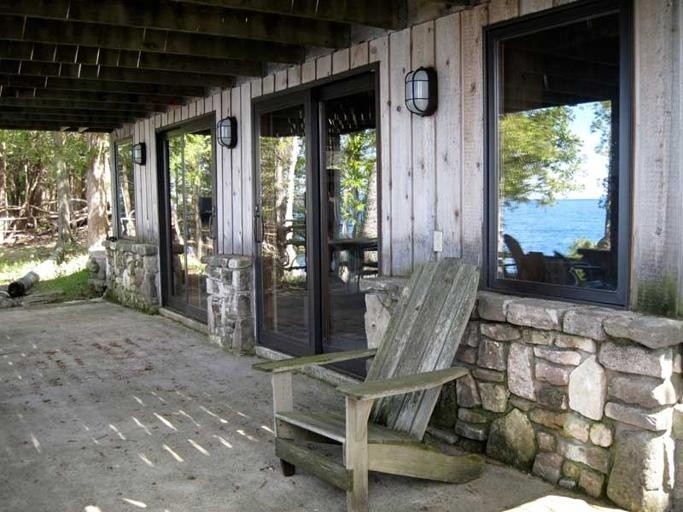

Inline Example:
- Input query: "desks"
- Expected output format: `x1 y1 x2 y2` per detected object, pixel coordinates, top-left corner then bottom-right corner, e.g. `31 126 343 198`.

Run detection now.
329 238 378 295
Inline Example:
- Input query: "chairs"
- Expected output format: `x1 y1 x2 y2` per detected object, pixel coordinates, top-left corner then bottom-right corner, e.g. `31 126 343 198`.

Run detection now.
503 233 577 287
273 217 307 290
250 255 487 512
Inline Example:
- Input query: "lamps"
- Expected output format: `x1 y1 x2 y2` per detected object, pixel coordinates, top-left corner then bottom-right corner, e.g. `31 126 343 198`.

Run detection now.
404 63 438 117
132 141 146 165
217 115 238 149
